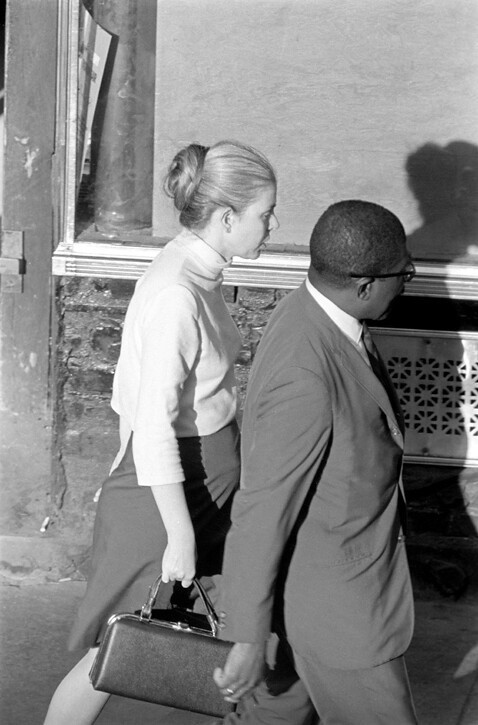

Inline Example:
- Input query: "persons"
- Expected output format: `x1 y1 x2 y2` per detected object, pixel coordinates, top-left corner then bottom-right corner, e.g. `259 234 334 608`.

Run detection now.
40 137 278 725
213 200 420 725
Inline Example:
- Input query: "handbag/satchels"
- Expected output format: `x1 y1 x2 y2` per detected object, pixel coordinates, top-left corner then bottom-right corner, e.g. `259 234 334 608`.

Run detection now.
87 573 237 719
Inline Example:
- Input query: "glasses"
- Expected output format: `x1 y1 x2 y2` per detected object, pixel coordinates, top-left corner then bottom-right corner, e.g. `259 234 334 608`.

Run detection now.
349 260 416 281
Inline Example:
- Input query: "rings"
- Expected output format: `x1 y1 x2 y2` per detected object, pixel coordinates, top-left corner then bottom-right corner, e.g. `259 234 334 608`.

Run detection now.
226 688 234 695
